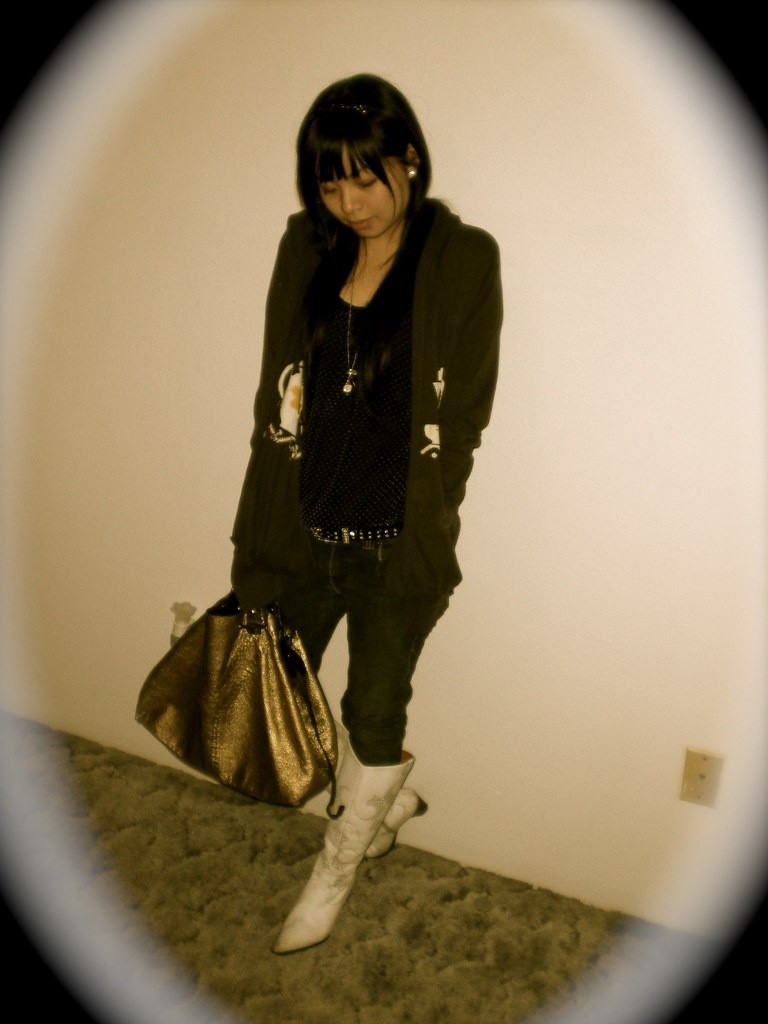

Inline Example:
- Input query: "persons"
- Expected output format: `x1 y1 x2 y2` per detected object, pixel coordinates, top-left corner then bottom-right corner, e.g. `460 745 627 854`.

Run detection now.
230 73 503 954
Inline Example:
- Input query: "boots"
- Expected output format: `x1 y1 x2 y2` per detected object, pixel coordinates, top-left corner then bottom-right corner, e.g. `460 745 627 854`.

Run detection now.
333 751 429 857
273 734 415 954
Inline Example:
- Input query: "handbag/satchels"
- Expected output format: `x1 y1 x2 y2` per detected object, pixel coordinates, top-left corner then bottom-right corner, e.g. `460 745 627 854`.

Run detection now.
135 591 339 808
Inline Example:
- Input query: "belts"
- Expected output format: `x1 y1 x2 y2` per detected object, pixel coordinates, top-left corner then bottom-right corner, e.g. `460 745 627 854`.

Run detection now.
306 526 401 544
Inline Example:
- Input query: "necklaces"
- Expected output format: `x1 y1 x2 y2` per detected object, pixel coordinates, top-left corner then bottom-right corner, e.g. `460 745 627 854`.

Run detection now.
343 270 358 394
